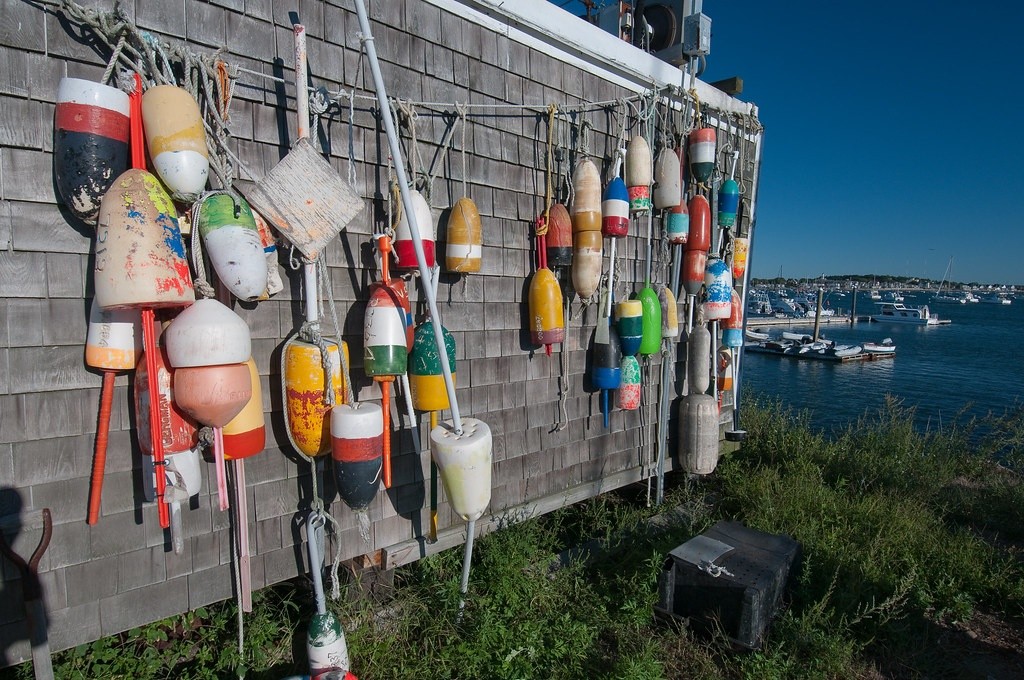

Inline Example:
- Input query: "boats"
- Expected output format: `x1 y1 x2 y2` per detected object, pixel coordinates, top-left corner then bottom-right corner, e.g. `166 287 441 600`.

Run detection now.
872 302 938 326
745 329 896 357
983 291 1012 305
748 282 982 318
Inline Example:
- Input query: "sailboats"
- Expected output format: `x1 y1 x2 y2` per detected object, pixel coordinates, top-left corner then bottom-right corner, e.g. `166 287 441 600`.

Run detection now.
929 256 965 304
